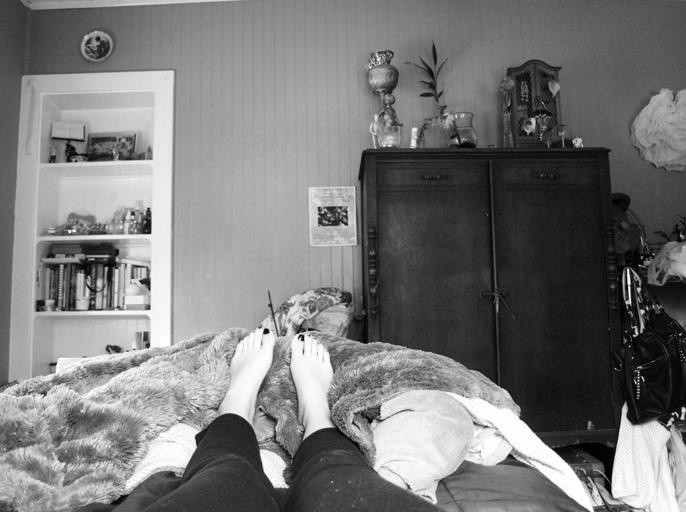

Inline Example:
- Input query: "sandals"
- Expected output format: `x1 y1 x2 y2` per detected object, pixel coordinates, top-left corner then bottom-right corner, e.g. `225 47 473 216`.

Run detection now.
574 468 607 512
589 469 629 512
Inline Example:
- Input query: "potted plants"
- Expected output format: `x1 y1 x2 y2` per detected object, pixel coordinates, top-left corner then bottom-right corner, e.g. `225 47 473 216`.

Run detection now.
416 111 452 148
401 40 477 149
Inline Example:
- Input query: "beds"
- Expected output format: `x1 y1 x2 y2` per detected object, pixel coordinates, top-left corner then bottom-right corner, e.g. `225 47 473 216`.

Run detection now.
2 326 582 511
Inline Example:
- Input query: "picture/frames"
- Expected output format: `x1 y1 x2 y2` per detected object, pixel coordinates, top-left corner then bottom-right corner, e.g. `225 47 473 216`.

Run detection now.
87 132 139 161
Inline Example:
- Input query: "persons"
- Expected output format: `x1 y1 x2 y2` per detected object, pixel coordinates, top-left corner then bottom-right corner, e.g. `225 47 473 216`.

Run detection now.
369 113 382 149
140 324 446 512
519 121 534 136
377 93 404 132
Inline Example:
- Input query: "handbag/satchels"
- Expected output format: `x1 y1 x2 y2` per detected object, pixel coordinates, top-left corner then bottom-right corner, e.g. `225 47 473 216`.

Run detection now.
614 263 686 425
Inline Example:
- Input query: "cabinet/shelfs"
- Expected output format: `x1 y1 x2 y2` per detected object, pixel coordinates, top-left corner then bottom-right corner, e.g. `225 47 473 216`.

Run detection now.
8 70 174 384
356 148 625 448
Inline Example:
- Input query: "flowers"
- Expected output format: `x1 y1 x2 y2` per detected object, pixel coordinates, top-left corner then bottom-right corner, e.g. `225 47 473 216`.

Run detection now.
628 87 686 173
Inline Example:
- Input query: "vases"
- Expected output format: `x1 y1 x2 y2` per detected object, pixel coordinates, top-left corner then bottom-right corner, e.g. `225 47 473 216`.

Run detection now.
409 127 427 149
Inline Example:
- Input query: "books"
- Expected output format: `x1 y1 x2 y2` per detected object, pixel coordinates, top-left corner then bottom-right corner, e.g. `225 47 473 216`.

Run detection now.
45 262 151 311
